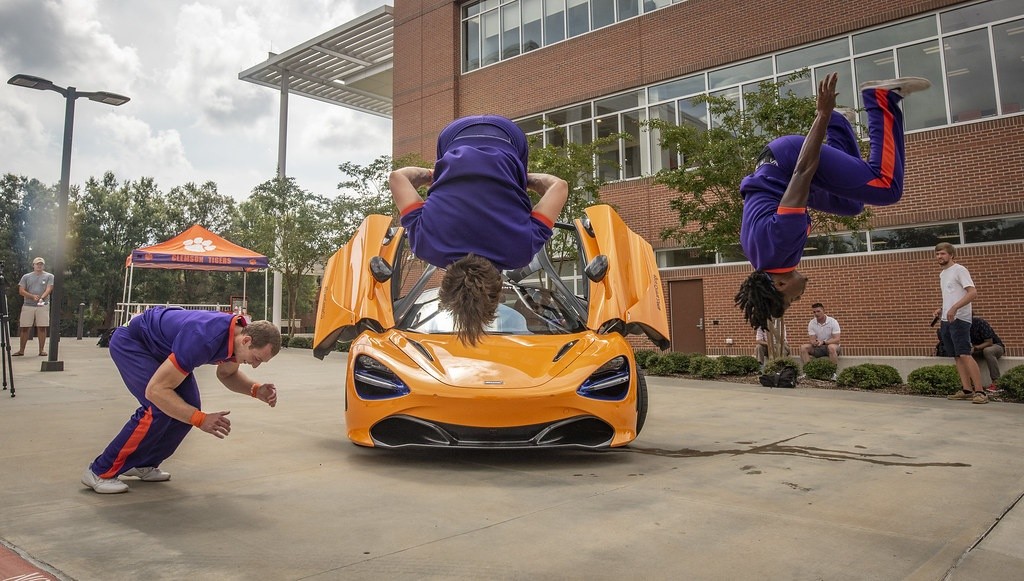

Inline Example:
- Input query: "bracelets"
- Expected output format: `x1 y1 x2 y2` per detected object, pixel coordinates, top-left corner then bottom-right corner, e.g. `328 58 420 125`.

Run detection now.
41 297 45 300
250 383 260 399
189 409 207 428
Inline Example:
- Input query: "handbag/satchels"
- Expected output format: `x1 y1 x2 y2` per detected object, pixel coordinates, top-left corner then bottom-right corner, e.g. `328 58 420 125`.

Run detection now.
759 365 798 387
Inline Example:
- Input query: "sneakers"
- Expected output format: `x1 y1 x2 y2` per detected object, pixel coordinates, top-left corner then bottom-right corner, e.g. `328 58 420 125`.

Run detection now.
814 107 857 122
121 466 171 481
79 462 129 493
947 389 975 399
859 77 931 98
972 391 989 404
986 383 1004 392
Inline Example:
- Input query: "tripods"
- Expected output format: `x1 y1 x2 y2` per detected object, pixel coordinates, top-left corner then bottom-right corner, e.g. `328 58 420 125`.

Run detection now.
0 260 15 397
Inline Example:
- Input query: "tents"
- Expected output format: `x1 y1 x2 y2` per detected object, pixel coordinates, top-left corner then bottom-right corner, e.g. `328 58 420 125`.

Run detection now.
120 226 270 326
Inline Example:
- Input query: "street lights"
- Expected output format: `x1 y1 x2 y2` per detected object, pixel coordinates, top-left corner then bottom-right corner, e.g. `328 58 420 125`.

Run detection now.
7 75 131 375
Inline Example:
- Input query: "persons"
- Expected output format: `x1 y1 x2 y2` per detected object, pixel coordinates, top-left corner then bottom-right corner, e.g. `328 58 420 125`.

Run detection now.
80 303 283 495
12 257 54 356
798 304 842 380
734 71 932 331
932 242 989 404
970 317 1006 392
388 113 569 349
756 319 792 365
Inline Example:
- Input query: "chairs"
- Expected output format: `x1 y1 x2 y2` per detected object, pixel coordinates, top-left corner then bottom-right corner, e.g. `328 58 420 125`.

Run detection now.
513 292 558 333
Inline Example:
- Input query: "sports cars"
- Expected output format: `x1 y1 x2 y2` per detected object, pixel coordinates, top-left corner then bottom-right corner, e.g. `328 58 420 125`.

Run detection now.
314 205 676 452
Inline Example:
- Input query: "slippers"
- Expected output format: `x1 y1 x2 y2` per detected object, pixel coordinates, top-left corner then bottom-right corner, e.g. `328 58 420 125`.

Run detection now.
38 351 47 356
11 351 24 356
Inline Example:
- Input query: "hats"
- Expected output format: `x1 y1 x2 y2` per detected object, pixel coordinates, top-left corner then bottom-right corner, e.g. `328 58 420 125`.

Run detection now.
32 257 45 264
